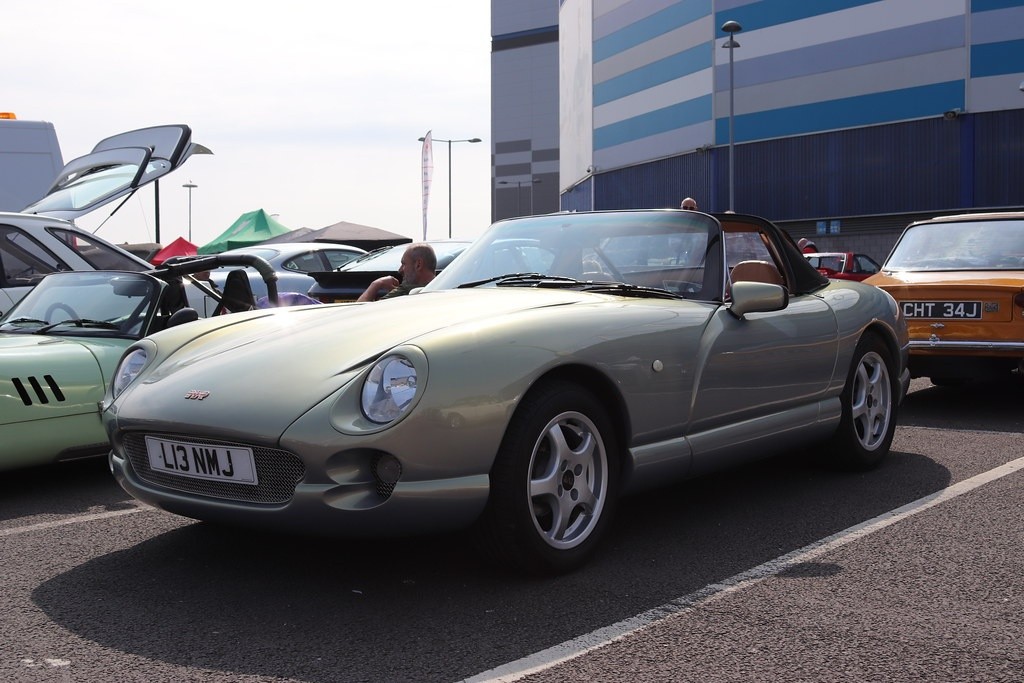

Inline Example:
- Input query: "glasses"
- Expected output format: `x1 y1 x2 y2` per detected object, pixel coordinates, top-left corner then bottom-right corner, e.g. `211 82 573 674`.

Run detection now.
683 206 694 210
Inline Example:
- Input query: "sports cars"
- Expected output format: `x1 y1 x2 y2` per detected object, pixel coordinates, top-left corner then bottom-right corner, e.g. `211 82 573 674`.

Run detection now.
0 110 779 486
96 207 912 584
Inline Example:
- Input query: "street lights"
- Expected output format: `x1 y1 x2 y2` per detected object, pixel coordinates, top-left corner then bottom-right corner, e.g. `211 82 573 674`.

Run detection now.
182 184 198 242
418 137 483 238
586 165 597 211
499 179 542 217
721 20 745 209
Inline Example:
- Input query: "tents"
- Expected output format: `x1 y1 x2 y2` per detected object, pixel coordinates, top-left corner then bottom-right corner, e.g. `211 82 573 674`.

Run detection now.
289 220 413 252
253 227 315 245
197 208 292 255
150 236 198 266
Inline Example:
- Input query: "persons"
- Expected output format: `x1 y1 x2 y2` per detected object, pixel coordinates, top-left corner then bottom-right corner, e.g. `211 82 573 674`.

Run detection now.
680 197 697 211
192 270 219 295
356 242 437 302
797 238 818 253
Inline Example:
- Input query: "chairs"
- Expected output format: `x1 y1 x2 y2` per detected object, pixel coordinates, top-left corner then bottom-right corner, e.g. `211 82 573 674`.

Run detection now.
731 260 783 295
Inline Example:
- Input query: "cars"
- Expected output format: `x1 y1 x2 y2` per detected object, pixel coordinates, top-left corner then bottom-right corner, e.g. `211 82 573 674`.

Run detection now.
860 211 1024 388
802 251 881 283
